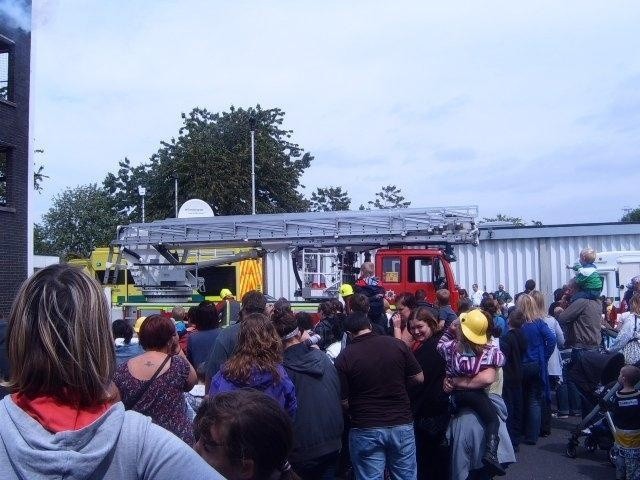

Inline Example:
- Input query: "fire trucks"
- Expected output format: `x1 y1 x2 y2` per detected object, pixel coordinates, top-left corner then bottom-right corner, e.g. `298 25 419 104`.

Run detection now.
100 202 480 337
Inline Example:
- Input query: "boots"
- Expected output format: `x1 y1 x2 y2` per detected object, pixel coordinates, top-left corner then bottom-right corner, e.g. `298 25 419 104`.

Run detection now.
481 433 506 476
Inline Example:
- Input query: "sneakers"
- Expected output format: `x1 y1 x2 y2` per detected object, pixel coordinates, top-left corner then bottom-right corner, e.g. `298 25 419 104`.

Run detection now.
551 413 569 419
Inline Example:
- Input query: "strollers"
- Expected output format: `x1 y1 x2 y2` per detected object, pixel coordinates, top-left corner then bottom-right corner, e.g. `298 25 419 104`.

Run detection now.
562 350 640 465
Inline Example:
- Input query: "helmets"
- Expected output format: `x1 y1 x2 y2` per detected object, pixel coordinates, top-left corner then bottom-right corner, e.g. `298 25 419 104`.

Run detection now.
220 289 232 299
339 284 354 297
134 316 147 333
460 309 488 345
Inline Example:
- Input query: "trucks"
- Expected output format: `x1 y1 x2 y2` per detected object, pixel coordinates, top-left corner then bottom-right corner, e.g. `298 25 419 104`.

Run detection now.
574 249 640 307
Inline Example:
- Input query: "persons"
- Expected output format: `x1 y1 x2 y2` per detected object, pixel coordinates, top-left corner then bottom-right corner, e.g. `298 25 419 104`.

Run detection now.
1 265 226 480
111 245 640 479
